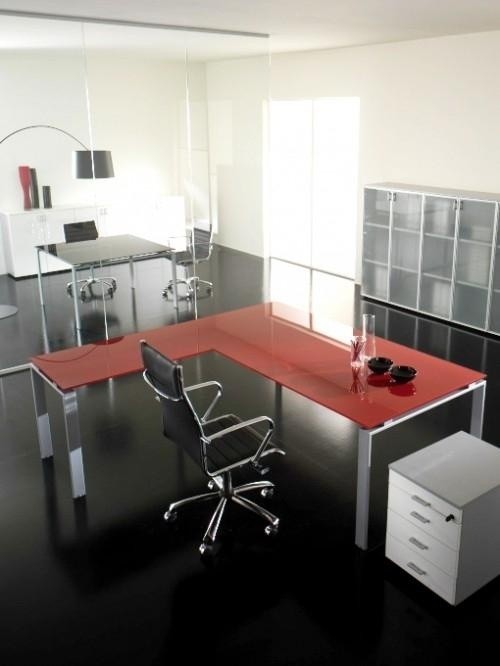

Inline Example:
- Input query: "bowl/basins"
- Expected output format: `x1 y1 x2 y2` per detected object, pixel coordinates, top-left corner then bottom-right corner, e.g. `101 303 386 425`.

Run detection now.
368 356 417 384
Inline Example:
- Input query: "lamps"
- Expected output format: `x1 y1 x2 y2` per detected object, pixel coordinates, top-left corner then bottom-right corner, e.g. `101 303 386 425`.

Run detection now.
0 125 113 179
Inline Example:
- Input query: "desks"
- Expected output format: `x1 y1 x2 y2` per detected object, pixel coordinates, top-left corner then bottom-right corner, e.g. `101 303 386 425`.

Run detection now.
29 302 487 549
35 234 179 354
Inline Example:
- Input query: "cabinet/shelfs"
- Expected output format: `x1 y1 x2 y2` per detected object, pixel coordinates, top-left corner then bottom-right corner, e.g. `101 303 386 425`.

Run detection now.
0 204 108 278
360 181 500 338
385 430 500 606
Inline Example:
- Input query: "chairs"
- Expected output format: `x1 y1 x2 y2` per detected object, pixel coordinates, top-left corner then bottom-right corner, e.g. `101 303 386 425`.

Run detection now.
63 221 116 302
140 340 286 555
161 224 214 302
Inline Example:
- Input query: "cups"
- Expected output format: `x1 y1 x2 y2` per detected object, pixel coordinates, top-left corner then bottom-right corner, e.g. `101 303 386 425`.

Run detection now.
350 314 375 367
